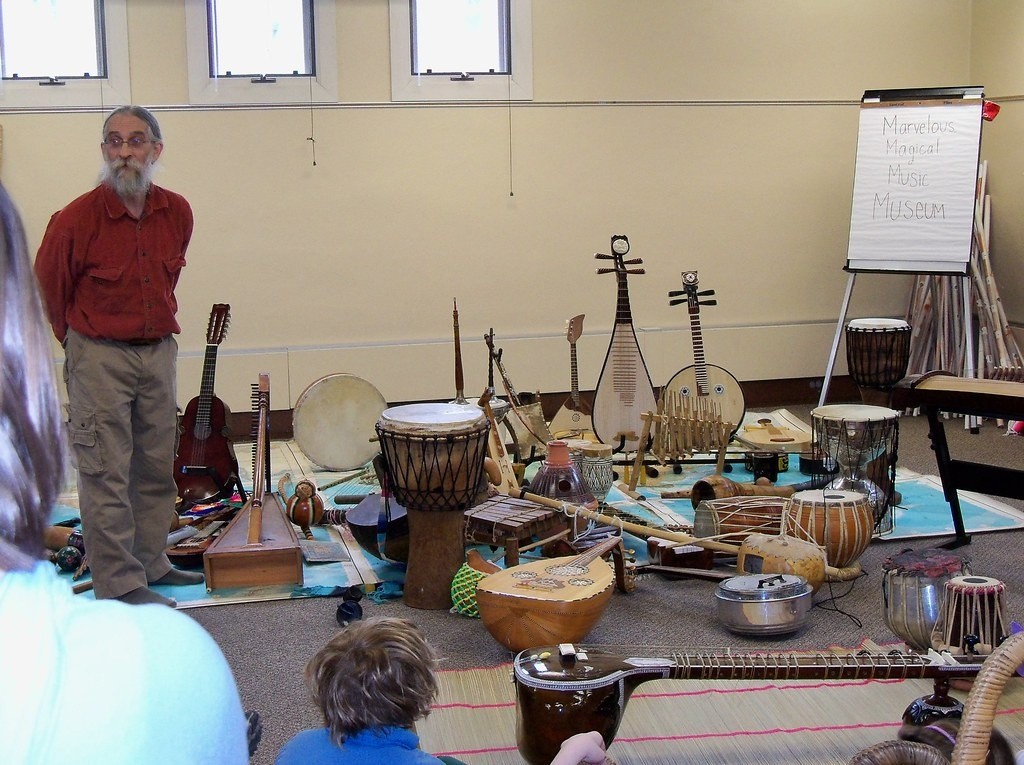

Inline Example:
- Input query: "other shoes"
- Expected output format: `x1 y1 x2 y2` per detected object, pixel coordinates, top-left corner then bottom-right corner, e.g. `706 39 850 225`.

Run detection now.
245 709 263 757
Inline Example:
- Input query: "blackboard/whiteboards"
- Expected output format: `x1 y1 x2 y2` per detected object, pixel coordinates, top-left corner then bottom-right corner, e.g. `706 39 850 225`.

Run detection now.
841 85 986 276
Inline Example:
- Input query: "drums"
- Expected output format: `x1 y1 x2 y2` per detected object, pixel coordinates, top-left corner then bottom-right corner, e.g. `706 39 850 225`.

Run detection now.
289 370 389 473
374 401 492 612
811 403 901 539
844 317 913 409
694 494 793 554
880 547 1012 695
787 487 876 568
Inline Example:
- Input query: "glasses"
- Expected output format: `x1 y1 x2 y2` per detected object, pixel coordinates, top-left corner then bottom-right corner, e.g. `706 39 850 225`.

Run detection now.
102 135 155 149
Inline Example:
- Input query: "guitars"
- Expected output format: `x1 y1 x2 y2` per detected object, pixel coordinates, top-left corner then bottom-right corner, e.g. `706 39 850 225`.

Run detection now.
173 299 240 504
547 312 594 436
474 535 640 657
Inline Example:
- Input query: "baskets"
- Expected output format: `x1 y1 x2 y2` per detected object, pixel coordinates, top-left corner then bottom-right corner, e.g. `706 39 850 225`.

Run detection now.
450 562 491 619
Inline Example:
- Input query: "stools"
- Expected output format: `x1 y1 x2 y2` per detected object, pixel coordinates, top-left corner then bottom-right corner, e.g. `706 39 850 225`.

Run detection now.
464 496 567 571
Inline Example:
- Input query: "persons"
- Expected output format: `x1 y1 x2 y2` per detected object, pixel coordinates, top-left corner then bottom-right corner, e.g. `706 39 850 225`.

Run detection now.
35 105 204 607
0 186 251 765
272 618 445 765
